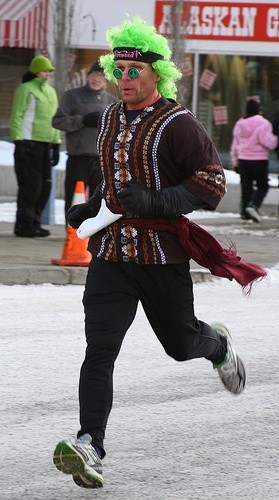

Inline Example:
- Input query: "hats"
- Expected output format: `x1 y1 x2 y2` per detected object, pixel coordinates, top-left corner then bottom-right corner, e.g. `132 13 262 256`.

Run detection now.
87 61 104 75
29 56 55 74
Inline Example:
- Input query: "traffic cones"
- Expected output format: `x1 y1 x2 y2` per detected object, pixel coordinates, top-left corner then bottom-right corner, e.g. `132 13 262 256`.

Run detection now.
52 180 93 267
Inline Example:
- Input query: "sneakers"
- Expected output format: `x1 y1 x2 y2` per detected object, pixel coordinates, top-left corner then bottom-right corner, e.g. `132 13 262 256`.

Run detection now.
53 433 104 489
212 322 246 395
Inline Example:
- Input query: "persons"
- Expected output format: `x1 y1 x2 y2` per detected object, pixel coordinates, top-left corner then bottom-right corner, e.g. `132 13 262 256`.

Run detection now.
51 19 247 490
230 93 278 224
8 55 120 239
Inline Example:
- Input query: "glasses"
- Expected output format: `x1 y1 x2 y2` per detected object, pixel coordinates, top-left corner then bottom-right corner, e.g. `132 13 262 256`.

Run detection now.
112 66 152 80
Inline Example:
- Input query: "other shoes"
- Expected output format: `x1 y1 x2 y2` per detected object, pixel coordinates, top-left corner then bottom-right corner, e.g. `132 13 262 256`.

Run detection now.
242 217 254 224
246 206 261 223
15 226 51 238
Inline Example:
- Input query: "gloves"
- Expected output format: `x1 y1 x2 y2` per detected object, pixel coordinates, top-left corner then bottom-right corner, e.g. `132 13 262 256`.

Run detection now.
235 167 241 173
65 196 100 229
117 181 162 217
50 144 59 166
14 141 26 162
83 111 101 125
